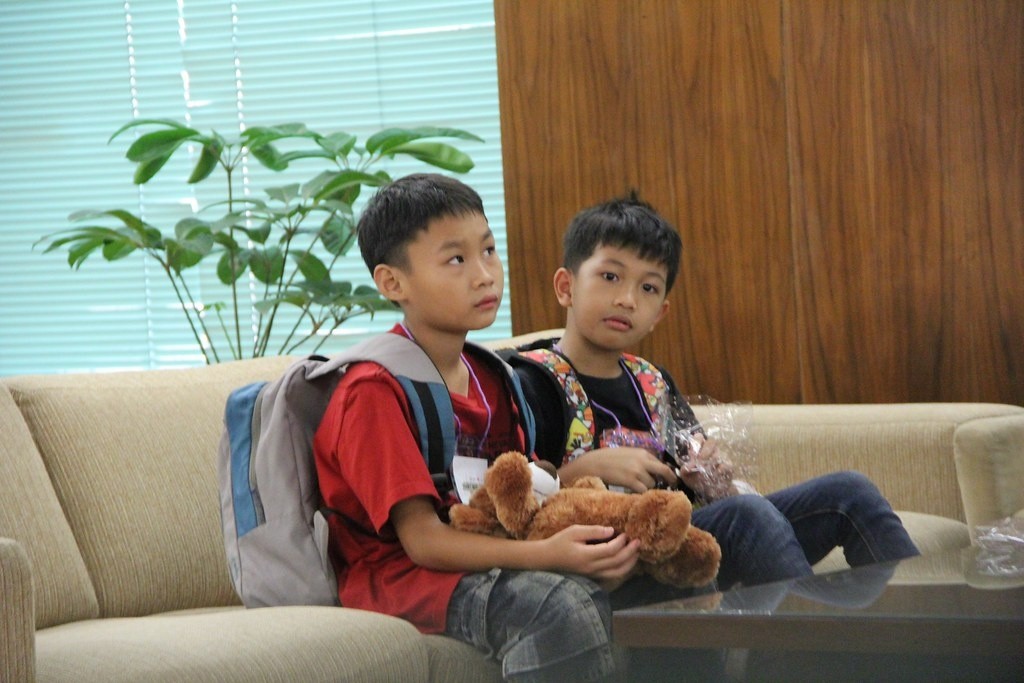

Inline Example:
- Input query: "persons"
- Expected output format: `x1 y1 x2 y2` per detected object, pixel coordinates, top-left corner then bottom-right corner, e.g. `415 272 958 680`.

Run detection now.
314 173 750 683
512 186 923 585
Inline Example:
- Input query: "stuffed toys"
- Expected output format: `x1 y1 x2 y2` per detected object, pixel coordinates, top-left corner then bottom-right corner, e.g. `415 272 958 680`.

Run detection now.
448 452 722 586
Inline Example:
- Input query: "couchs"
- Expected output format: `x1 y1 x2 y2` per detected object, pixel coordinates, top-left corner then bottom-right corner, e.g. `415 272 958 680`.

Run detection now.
1 328 1024 683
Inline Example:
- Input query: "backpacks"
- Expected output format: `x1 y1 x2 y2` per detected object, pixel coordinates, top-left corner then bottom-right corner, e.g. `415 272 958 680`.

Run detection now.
218 333 538 609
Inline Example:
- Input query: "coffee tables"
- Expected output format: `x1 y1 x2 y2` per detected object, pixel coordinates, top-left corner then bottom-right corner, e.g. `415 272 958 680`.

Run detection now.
613 555 1024 683
